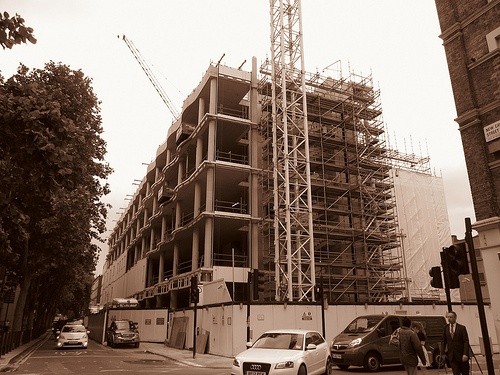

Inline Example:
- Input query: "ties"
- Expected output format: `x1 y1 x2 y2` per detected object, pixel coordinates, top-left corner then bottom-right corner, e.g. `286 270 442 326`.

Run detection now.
451 324 454 332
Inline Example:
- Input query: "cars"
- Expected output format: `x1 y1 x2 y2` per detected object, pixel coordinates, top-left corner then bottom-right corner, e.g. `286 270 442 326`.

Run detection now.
56 325 91 349
231 329 334 375
106 320 141 348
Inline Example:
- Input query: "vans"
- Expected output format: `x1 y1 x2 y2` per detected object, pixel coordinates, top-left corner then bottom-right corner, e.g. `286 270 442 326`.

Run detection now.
330 314 448 373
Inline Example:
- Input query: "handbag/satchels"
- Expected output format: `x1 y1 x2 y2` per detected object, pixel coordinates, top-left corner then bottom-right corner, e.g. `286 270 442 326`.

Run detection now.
388 328 400 346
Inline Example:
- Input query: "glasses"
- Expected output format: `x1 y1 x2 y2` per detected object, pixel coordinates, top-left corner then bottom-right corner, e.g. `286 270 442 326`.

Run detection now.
446 315 453 318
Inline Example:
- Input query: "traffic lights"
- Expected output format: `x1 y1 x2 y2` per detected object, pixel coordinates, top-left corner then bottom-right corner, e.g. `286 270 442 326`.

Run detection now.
429 267 442 288
449 242 471 289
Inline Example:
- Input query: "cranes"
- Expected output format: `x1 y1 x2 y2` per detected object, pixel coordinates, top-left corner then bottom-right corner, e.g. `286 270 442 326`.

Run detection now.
122 35 181 120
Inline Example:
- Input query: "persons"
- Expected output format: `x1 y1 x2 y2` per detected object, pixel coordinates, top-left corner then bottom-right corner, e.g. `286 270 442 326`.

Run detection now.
398 318 430 375
440 312 470 375
